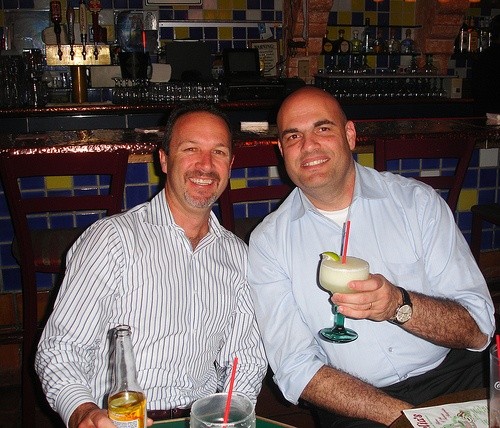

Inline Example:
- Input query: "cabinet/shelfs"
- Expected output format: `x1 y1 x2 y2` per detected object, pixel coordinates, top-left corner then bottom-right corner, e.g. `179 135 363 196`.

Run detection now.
317 24 458 78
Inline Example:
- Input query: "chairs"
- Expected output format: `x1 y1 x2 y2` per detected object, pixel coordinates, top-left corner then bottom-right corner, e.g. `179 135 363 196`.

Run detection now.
0 138 499 428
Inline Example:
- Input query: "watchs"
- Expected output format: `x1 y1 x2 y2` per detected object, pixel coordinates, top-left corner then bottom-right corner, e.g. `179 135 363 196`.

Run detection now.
387 286 413 326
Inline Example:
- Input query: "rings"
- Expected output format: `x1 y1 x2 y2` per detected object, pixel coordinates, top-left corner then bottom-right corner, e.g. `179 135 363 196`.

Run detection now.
369 302 372 310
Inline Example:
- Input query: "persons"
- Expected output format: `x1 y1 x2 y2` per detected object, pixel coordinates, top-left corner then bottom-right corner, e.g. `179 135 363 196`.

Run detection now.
247 88 496 428
34 100 268 428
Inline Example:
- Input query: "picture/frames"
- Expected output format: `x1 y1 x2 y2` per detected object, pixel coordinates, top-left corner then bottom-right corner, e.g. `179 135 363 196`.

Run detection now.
248 40 280 78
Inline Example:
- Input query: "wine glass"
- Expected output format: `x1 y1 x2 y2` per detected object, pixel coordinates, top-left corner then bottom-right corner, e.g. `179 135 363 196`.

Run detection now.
317 76 448 99
113 76 225 105
316 253 370 344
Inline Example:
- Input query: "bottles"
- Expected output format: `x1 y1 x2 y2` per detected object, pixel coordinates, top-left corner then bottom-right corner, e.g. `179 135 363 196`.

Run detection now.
0 50 47 112
321 27 437 74
456 12 500 55
107 324 146 428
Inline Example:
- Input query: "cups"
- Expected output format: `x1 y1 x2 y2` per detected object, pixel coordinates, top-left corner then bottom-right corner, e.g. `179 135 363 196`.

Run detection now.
489 342 500 428
189 391 257 428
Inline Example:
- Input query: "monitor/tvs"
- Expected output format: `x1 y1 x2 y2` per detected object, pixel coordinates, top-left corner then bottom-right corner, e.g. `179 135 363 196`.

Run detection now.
222 48 260 76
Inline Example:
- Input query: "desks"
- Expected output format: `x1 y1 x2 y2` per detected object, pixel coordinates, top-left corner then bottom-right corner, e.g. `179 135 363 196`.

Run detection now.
389 387 489 428
